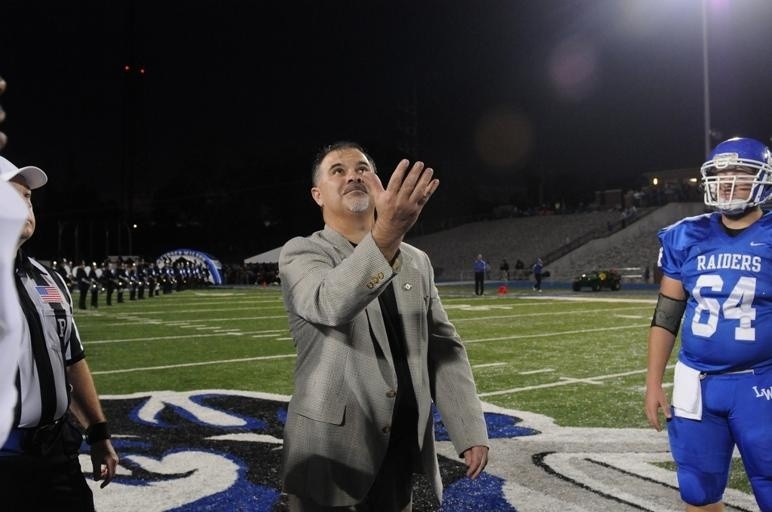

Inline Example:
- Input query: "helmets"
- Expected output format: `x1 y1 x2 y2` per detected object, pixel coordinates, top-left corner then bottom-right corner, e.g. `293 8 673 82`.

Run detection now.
700 135 772 217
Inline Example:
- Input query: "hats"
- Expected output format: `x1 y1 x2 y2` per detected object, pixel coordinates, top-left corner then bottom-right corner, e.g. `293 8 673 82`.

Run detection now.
0 155 49 190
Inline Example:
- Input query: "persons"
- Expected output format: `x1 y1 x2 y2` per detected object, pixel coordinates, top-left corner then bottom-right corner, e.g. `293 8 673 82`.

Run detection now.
53 251 284 311
514 259 527 280
641 134 771 511
472 254 485 296
277 141 491 511
500 258 509 280
531 258 544 292
0 154 121 510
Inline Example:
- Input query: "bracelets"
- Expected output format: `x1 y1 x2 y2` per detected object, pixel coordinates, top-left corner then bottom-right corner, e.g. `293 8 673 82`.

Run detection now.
84 422 112 444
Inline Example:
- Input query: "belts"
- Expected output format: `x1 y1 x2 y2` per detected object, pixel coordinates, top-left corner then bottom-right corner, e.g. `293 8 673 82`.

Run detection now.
20 414 65 451
